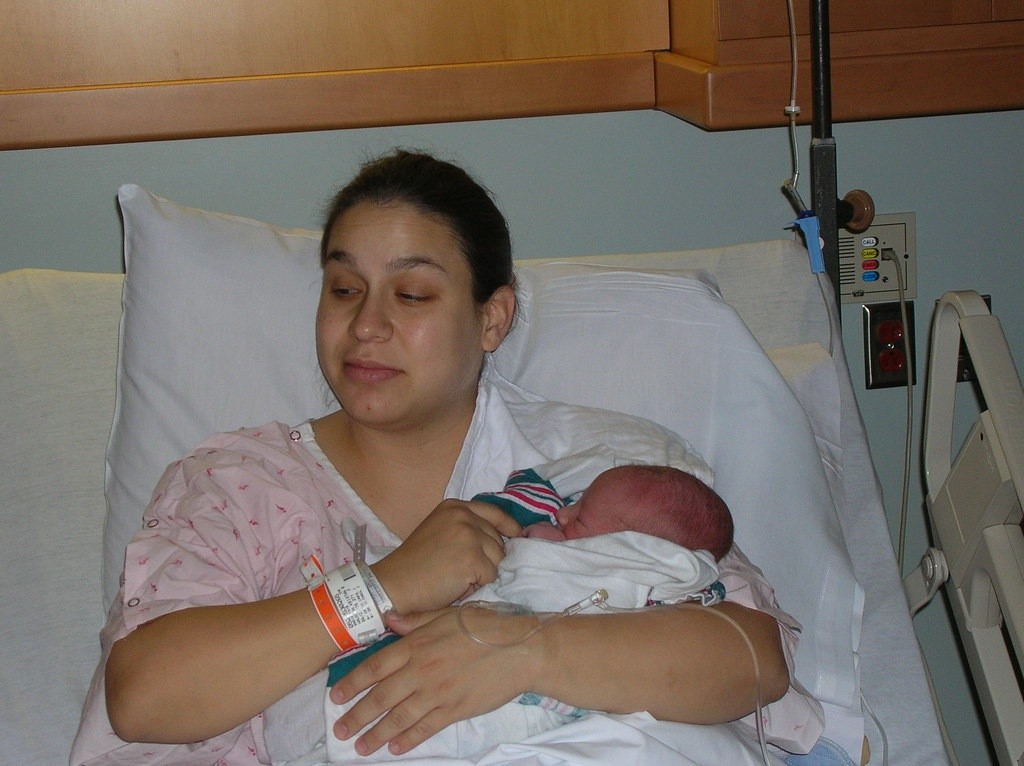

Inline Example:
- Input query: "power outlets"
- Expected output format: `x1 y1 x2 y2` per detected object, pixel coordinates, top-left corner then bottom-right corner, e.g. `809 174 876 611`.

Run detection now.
935 294 992 383
862 300 918 390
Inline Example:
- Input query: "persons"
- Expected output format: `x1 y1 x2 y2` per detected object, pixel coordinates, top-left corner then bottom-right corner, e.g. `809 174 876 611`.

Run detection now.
264 460 734 766
103 147 795 766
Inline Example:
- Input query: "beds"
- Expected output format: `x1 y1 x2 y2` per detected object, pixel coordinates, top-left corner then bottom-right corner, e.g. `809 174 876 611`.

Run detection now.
0 238 1024 766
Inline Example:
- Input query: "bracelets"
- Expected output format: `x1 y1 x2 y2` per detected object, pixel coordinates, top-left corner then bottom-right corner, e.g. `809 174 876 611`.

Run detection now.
299 524 400 654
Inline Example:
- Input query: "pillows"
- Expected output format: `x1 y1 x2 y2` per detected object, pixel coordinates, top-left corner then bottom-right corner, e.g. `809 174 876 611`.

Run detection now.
103 185 873 766
